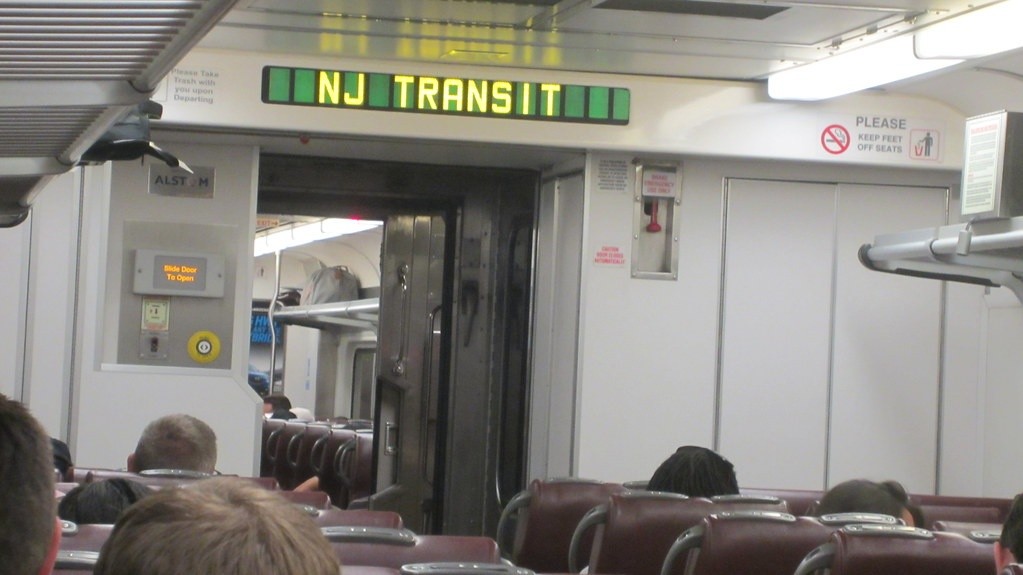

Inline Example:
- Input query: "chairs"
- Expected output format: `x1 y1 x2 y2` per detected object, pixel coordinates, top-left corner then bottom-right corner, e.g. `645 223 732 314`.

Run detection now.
47 414 1010 575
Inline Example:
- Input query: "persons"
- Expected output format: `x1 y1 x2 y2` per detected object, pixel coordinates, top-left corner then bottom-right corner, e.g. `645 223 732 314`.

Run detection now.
815 481 924 531
577 445 740 575
344 418 373 429
1 394 344 575
994 494 1023 575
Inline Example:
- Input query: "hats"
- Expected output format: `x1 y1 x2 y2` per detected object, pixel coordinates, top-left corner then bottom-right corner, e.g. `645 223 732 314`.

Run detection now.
49 439 73 469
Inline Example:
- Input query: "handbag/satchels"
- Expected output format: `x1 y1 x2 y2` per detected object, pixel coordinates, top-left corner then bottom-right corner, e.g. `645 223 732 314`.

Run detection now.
300 265 358 305
